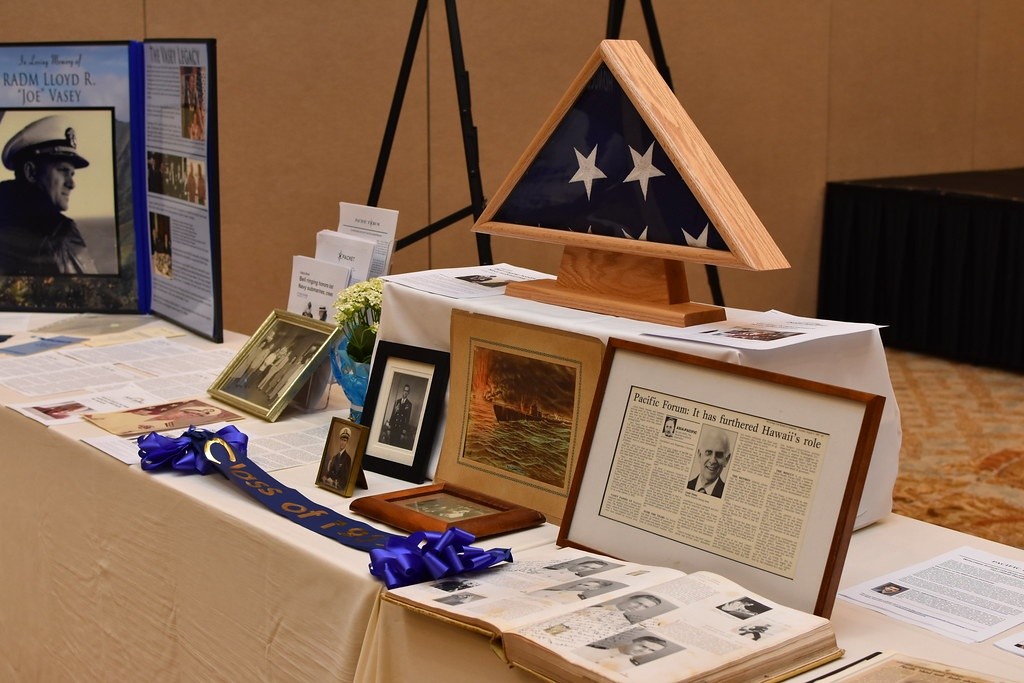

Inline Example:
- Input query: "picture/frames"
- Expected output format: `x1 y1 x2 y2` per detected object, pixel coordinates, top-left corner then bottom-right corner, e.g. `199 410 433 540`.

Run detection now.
359 339 451 485
557 335 887 620
146 35 223 343
206 308 342 423
314 416 369 497
0 40 144 315
349 483 547 538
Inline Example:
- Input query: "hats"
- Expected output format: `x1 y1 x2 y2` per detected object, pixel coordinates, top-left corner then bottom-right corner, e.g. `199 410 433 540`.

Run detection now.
339 427 352 438
2 113 90 170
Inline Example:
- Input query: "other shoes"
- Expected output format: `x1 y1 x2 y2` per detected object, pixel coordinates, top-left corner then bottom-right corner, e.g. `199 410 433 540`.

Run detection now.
223 385 251 390
264 391 271 406
256 386 262 391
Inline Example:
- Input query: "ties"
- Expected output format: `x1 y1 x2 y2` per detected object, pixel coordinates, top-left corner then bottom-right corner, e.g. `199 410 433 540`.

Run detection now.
335 453 340 472
403 399 404 403
700 489 705 493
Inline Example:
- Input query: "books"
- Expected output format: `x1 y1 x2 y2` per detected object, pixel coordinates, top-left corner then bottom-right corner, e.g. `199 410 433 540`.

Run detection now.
379 546 845 683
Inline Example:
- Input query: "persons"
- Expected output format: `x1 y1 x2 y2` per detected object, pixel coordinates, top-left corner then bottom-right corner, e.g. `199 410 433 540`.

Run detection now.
0 113 98 275
185 162 206 206
723 600 758 618
530 580 616 606
551 560 608 579
664 417 674 436
322 427 352 491
149 230 159 256
875 584 901 595
387 384 412 446
231 339 306 402
584 595 661 632
34 401 87 421
92 405 221 434
687 428 732 499
181 69 205 138
574 636 670 675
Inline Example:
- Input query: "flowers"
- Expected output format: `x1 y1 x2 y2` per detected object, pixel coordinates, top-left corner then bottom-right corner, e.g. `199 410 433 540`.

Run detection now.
327 277 385 364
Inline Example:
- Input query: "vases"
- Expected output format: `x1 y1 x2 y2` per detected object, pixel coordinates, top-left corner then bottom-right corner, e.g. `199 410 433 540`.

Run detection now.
328 322 371 425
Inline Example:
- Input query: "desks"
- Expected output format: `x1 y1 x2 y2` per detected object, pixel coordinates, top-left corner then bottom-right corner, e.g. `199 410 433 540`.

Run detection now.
1 312 1023 683
